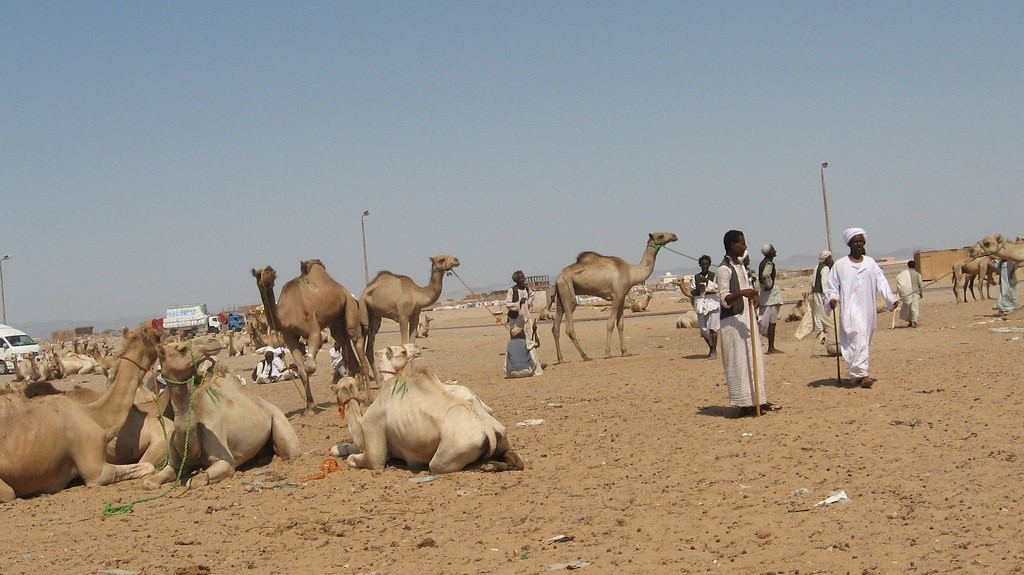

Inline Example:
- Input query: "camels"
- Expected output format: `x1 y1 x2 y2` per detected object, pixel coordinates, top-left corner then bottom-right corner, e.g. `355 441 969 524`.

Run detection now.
0 317 304 503
246 254 525 474
952 233 1024 303
631 293 652 312
548 231 677 364
877 291 887 313
671 278 699 329
538 306 555 320
785 292 815 322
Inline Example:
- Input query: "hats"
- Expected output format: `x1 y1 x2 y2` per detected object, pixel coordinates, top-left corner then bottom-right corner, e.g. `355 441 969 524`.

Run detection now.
760 244 771 256
818 249 831 262
843 228 867 246
275 347 284 357
512 327 523 338
738 251 749 265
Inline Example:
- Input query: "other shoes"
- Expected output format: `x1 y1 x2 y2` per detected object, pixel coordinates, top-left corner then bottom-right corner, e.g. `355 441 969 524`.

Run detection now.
761 402 774 411
860 376 873 388
739 404 765 416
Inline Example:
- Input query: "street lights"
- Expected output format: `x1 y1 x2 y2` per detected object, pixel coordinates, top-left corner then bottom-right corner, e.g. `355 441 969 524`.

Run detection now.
820 161 833 261
361 210 372 288
0 254 12 325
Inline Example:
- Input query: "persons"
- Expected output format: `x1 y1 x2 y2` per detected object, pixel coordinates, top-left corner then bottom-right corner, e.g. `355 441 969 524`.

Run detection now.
252 347 300 384
505 318 540 378
810 251 842 358
827 228 899 388
757 243 784 354
328 341 343 367
505 270 548 369
714 230 782 416
992 259 1020 312
690 255 721 359
738 250 757 289
896 260 923 327
13 336 24 345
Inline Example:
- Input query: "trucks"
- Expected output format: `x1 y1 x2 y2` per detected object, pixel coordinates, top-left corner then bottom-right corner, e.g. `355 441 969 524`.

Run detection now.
162 303 223 336
226 312 257 332
0 324 41 375
